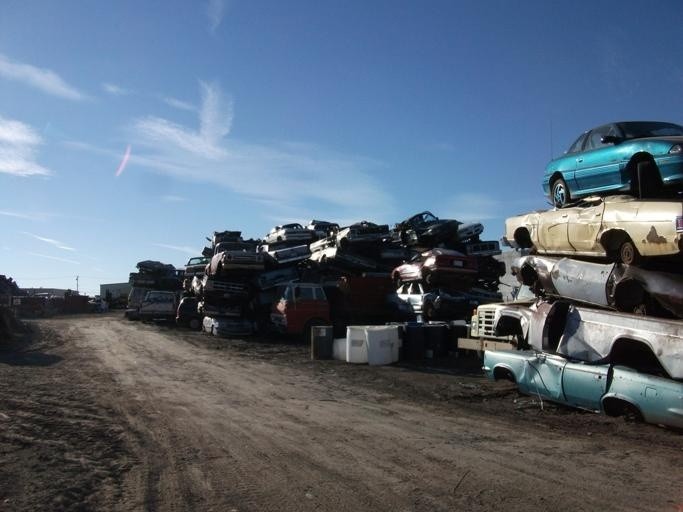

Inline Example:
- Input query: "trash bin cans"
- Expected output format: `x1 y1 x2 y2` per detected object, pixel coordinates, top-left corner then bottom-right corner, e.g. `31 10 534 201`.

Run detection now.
404 326 459 370
310 326 334 360
